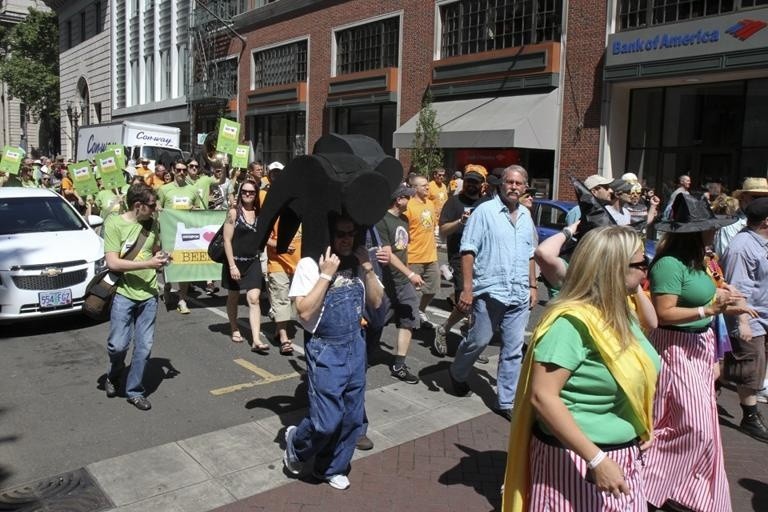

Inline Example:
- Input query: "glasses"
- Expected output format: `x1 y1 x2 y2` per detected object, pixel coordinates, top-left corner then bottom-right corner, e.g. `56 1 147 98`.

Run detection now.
144 203 156 209
189 165 198 168
629 255 650 270
336 230 357 238
623 190 630 194
400 195 410 200
176 169 187 172
240 190 256 196
596 185 608 191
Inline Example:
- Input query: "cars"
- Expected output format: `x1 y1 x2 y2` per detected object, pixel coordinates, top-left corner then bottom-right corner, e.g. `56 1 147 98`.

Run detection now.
0 186 111 326
527 197 660 290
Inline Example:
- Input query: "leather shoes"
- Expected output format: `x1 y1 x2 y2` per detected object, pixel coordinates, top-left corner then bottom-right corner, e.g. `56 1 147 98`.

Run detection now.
739 413 767 443
104 374 122 398
355 435 373 449
493 409 512 421
448 363 468 398
127 394 151 410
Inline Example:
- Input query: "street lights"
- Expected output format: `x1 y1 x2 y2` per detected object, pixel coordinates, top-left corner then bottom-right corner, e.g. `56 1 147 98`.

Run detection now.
65 98 85 164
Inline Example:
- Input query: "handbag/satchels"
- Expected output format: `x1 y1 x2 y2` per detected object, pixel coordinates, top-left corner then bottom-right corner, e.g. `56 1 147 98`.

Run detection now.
207 207 240 262
80 269 121 320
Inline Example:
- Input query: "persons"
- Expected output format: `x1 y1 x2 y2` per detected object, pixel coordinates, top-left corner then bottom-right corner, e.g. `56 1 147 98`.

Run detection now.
1 157 303 352
537 173 768 442
642 192 759 512
357 162 536 384
104 185 173 410
498 224 663 512
445 161 539 422
281 213 383 489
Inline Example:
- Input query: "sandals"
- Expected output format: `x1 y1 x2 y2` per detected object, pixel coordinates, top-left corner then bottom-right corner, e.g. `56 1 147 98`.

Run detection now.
231 328 243 342
279 342 293 353
251 342 269 352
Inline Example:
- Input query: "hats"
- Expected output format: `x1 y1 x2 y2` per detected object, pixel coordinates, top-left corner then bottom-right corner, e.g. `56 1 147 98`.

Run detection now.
463 171 482 183
584 174 615 190
268 161 285 171
611 179 633 192
653 177 767 234
397 183 416 197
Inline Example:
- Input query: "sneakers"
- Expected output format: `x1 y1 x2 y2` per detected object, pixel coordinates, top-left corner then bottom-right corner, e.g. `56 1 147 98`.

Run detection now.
312 470 350 490
205 282 219 294
283 424 305 475
162 290 171 304
418 309 433 328
390 363 418 384
177 299 190 314
476 353 489 364
434 325 449 356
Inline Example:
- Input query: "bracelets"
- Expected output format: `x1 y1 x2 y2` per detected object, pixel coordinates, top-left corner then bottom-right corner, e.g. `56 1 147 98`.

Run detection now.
587 450 608 469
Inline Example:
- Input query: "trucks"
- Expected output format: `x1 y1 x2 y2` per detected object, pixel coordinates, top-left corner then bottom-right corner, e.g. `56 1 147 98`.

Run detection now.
76 121 187 175
